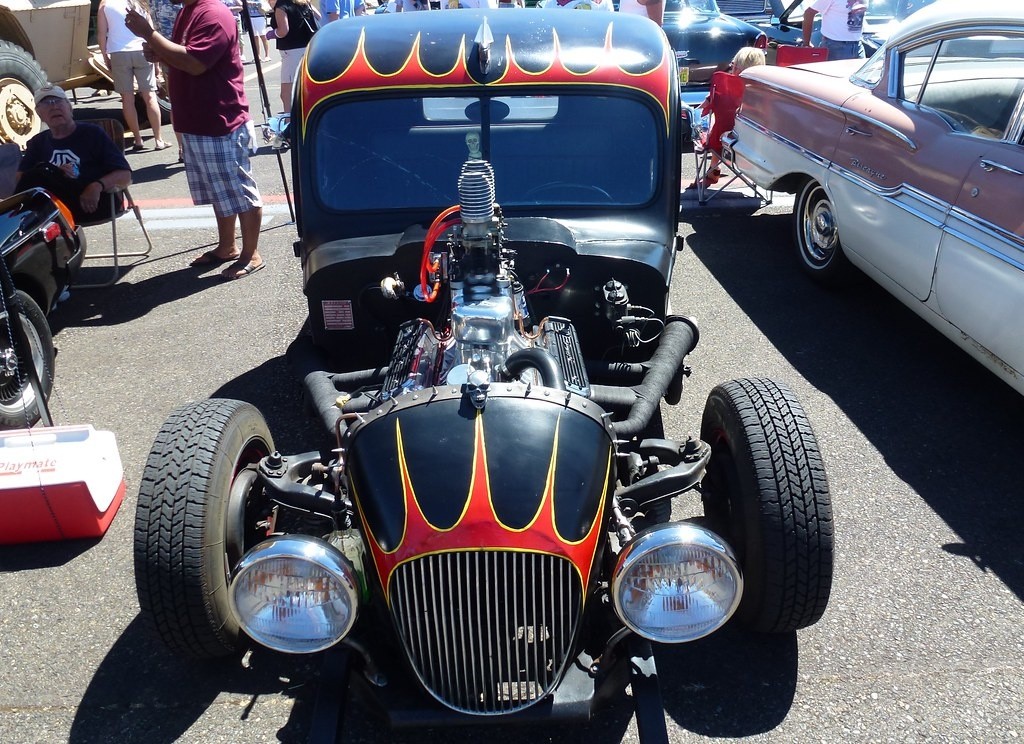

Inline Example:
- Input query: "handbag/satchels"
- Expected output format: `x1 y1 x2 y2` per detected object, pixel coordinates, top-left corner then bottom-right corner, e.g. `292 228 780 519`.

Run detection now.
306 0 322 39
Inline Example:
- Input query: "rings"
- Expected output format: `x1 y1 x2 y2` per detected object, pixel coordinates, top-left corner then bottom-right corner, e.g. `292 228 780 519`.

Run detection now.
90 204 94 207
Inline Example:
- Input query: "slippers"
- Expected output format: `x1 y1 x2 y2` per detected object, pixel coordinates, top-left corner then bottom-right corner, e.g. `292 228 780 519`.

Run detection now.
133 139 144 147
223 260 266 280
155 142 172 150
189 252 240 267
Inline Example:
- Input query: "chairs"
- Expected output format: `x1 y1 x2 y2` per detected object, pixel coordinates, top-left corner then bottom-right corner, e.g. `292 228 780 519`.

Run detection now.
67 117 151 291
524 109 651 203
686 84 773 208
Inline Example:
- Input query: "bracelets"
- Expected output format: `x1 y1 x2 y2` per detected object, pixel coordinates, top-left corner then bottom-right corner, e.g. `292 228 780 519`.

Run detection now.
93 179 105 192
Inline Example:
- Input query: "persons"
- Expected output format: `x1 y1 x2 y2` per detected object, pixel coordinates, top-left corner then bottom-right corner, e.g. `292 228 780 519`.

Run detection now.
247 0 272 63
96 0 173 151
680 47 767 189
318 0 429 27
265 0 322 126
544 0 666 28
149 0 187 163
802 0 868 60
12 85 133 311
125 0 267 281
220 0 247 60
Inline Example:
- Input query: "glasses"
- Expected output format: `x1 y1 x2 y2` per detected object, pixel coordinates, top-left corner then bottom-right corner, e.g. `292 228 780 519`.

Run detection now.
36 98 66 107
729 61 742 70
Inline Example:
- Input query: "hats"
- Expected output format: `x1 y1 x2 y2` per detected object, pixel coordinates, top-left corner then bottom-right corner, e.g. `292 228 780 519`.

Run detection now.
34 86 66 105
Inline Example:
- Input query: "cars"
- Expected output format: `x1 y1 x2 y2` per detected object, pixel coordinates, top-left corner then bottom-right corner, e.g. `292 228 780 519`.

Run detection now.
742 0 953 65
661 0 769 82
0 188 90 431
716 0 1023 395
134 4 837 736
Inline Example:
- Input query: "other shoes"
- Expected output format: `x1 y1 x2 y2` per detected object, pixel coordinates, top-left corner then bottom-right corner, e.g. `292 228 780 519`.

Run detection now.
263 57 271 62
252 57 261 62
240 55 246 60
